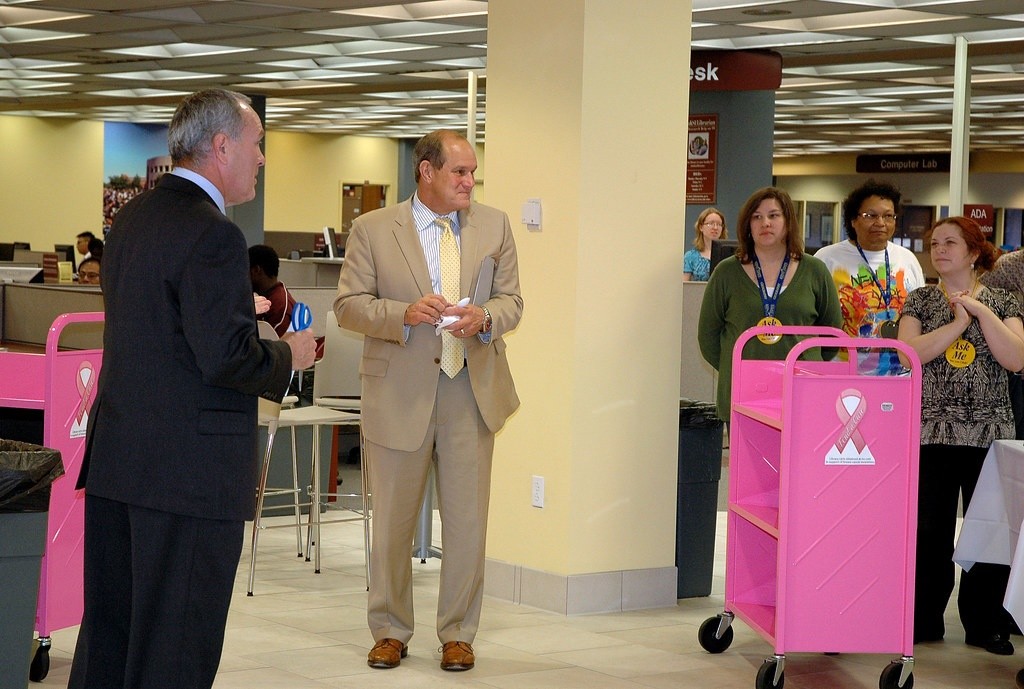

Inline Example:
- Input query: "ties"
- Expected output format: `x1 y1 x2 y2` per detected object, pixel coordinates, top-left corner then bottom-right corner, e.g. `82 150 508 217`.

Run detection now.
433 217 464 379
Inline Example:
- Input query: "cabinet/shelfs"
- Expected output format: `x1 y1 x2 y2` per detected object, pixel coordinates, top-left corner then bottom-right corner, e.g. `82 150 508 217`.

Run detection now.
341 184 388 233
0 311 106 681
699 326 918 689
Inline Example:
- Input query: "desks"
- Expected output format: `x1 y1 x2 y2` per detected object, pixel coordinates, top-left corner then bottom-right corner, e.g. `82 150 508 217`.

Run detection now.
1 244 106 354
951 438 1024 638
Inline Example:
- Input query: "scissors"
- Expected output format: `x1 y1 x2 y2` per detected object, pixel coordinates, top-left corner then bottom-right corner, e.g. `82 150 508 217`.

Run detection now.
292 302 312 392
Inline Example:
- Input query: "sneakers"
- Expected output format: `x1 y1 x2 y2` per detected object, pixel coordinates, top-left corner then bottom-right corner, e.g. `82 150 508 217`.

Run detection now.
965 632 1014 656
913 627 944 644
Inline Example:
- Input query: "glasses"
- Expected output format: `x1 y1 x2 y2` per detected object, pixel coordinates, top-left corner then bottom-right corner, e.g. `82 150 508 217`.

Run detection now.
78 240 90 244
703 222 723 229
858 211 898 223
77 272 100 282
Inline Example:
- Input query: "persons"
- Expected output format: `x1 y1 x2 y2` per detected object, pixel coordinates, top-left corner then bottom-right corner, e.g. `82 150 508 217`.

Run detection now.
684 208 728 281
978 249 1024 637
333 130 523 671
697 186 845 445
247 245 300 338
74 231 105 284
68 89 318 689
896 216 1024 655
813 180 925 378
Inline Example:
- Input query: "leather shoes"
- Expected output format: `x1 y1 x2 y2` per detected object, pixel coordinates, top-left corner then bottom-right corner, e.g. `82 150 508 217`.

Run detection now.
438 642 473 669
368 638 408 668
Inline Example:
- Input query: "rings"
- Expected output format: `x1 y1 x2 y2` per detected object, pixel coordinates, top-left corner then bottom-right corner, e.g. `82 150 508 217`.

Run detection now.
461 329 464 335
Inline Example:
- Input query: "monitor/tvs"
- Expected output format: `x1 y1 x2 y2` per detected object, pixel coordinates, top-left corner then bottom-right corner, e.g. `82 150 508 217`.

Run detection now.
0 242 44 284
710 239 740 276
54 245 78 273
323 226 338 258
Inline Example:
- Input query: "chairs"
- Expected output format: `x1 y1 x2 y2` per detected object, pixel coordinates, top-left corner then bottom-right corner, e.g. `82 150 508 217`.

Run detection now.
246 311 372 596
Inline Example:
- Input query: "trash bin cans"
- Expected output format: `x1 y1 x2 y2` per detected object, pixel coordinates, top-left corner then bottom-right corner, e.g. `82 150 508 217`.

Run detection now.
675 401 724 598
0 440 61 689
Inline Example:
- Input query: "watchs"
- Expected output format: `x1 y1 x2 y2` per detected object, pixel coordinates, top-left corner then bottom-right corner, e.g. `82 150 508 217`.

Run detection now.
477 305 492 336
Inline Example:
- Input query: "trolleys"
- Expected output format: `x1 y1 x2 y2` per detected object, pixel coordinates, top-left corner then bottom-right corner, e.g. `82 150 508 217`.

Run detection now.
0 310 106 683
697 326 923 688
